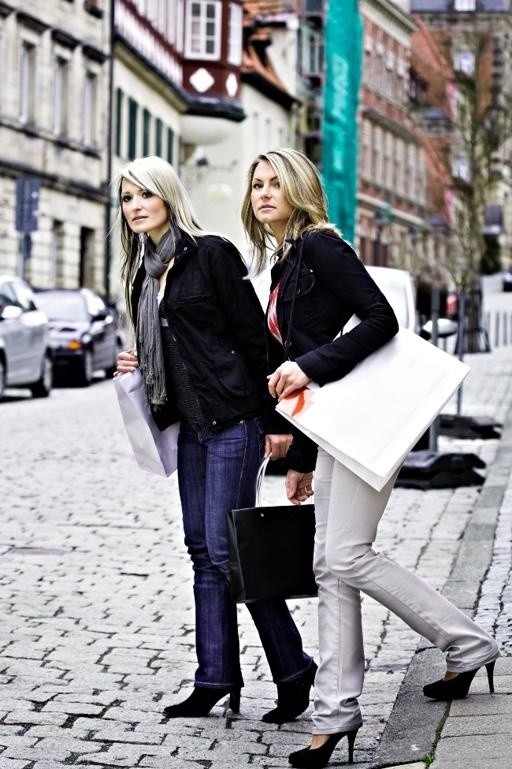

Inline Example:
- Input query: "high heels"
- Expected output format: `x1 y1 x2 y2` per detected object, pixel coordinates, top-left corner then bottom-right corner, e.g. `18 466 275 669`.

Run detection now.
161 677 242 719
422 658 497 701
261 662 319 724
288 720 359 768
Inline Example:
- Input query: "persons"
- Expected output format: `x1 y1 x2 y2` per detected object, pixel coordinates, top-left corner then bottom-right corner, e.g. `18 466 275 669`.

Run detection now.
240 145 501 769
115 156 318 720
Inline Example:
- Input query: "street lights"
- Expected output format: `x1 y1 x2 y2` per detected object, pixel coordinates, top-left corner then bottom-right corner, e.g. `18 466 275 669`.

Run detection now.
423 104 468 458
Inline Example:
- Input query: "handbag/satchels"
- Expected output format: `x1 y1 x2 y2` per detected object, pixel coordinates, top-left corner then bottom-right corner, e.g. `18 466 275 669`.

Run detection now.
273 225 473 494
225 453 319 605
112 366 180 479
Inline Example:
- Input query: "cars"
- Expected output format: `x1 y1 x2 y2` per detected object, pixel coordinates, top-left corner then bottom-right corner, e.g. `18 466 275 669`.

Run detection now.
33 288 125 388
0 272 55 401
